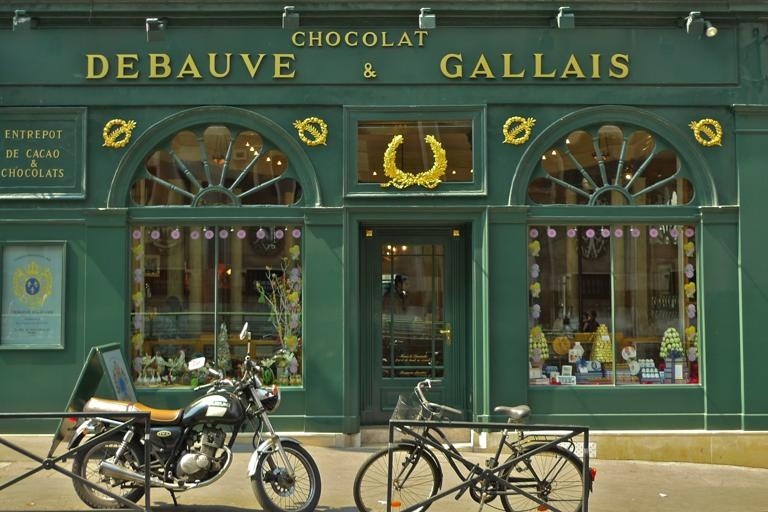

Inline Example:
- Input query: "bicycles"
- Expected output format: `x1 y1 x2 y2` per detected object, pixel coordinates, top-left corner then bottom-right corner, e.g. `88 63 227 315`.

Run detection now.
353 375 598 512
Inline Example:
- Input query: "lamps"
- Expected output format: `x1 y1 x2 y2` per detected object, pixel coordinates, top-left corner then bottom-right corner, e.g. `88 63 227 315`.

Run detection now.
10 6 36 34
277 4 302 33
416 6 439 32
680 7 717 38
141 11 172 39
553 5 575 30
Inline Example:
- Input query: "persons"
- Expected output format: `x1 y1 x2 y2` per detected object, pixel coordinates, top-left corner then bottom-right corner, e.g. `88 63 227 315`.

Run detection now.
152 297 182 336
383 274 410 376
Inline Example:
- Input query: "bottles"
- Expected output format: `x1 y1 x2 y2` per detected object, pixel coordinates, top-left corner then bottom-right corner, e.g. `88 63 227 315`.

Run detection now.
638 358 659 378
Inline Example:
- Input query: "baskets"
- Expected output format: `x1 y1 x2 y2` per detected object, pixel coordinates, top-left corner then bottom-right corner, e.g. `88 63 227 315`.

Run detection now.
391 394 421 429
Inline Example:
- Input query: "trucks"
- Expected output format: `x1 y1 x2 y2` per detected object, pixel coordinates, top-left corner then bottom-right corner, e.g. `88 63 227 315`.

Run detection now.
569 331 662 371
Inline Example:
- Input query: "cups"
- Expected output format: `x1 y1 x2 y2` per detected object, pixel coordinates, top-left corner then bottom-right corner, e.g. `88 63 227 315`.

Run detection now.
550 371 560 383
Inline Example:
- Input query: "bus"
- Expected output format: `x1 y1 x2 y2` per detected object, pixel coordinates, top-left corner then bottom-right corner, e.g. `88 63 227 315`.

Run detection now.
143 334 281 374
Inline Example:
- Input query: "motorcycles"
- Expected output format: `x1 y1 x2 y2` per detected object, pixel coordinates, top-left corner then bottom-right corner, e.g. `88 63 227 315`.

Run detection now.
60 320 322 512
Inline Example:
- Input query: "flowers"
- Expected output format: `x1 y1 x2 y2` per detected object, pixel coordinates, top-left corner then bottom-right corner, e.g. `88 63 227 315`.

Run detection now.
529 326 550 360
255 248 301 356
660 326 684 358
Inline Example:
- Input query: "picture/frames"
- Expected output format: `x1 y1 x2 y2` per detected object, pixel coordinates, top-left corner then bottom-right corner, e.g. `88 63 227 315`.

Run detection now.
0 237 69 354
0 102 91 203
